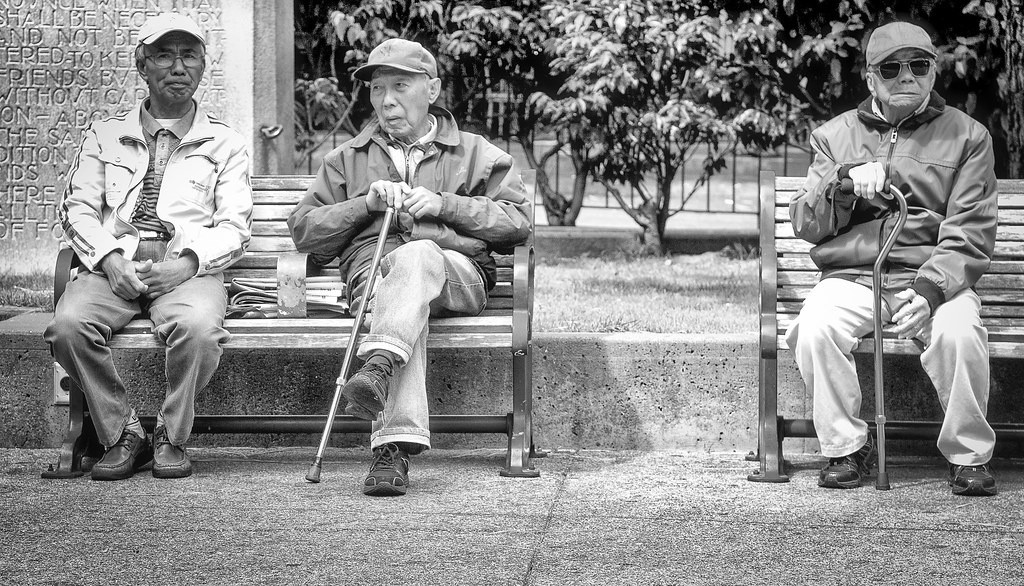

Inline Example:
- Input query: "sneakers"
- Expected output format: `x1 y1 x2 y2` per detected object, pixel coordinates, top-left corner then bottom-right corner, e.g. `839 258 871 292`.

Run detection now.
341 362 389 421
362 442 410 496
152 424 193 479
947 460 997 495
91 425 152 481
818 430 877 489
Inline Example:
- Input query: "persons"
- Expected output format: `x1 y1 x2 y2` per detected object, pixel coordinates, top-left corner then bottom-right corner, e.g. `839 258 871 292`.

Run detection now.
45 13 254 480
786 20 998 496
287 38 534 496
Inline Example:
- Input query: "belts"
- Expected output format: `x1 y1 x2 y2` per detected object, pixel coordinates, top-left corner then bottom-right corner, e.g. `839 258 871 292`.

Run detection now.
138 228 172 242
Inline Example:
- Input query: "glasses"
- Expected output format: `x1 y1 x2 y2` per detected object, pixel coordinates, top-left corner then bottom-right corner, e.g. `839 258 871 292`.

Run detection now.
145 52 203 69
872 57 935 80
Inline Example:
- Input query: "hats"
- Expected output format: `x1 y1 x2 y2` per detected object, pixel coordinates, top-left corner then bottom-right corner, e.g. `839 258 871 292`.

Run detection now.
134 13 206 53
352 38 438 81
865 21 938 67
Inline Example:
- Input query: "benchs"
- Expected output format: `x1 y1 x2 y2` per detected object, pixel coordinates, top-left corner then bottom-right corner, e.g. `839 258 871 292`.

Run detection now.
40 169 548 478
745 171 1024 482
